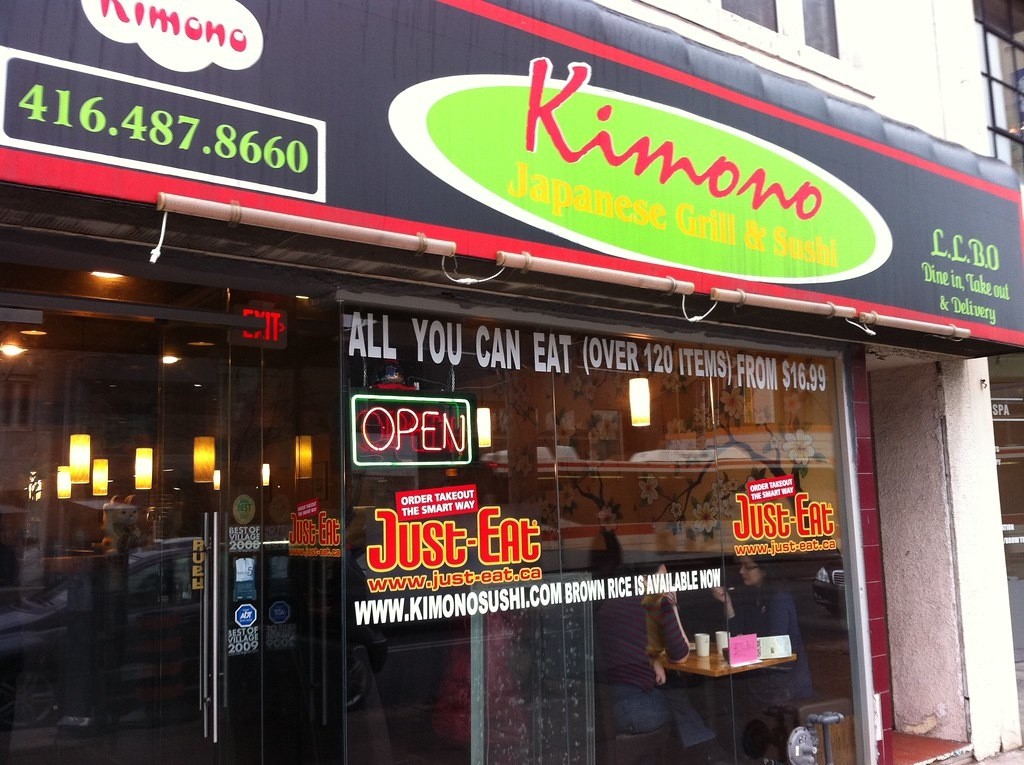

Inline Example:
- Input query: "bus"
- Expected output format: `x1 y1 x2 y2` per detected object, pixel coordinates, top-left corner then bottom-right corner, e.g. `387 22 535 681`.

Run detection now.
350 446 842 556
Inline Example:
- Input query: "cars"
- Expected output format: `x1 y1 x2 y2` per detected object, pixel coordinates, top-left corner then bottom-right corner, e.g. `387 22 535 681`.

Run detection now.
810 559 847 617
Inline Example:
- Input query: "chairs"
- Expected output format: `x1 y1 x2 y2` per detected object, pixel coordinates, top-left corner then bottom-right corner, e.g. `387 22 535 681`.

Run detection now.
507 625 687 763
286 632 472 765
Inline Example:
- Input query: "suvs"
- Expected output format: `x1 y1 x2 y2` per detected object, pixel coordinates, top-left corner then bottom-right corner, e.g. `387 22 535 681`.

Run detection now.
1 536 388 730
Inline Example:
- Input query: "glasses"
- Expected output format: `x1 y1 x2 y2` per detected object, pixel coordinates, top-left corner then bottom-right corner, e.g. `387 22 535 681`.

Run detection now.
735 562 760 572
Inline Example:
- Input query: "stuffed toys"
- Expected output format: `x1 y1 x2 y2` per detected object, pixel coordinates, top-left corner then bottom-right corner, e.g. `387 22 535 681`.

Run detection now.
100 494 143 556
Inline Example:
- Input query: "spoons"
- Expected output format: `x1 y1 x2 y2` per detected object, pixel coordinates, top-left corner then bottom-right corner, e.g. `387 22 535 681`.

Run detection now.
726 588 735 590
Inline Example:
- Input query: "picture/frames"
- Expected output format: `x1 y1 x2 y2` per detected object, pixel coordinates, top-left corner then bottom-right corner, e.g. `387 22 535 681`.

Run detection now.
311 461 329 502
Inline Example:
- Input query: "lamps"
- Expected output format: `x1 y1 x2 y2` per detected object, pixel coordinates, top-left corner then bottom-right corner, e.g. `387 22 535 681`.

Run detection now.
476 367 493 448
192 383 217 484
292 358 313 479
628 373 651 426
1 323 29 355
56 317 110 501
134 377 153 490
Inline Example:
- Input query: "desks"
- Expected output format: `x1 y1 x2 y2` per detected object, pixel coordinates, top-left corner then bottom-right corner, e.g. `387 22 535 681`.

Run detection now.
647 640 797 765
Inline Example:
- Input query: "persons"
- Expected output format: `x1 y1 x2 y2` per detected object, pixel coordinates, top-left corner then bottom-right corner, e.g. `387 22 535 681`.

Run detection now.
593 553 728 765
712 540 815 765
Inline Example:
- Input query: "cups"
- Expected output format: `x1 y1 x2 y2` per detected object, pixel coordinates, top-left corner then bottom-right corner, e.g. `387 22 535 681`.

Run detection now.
694 633 710 657
715 631 730 654
